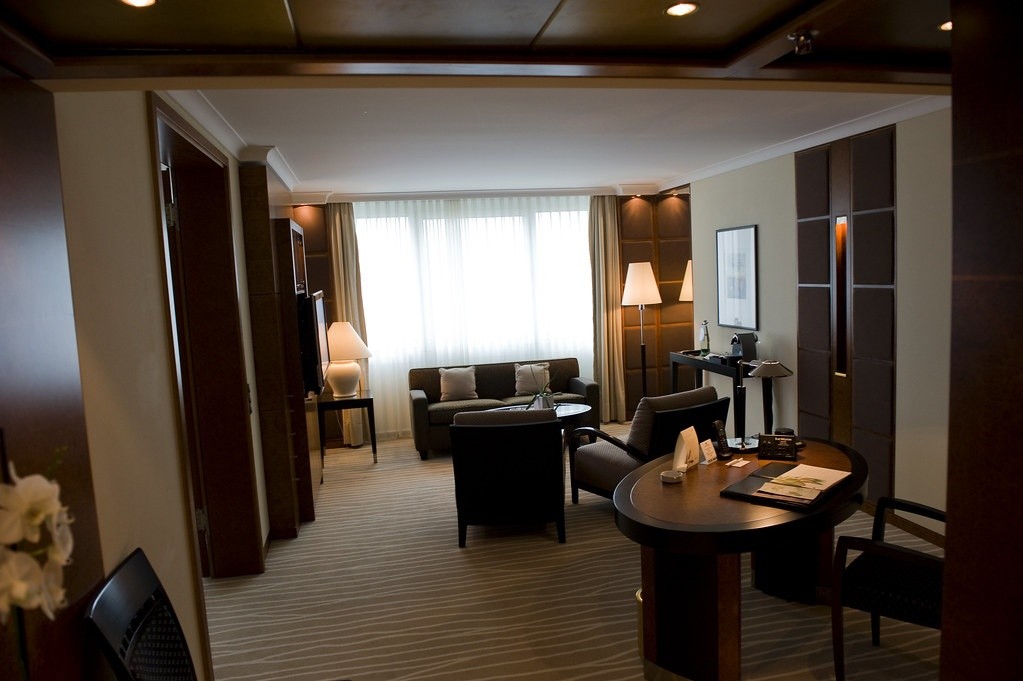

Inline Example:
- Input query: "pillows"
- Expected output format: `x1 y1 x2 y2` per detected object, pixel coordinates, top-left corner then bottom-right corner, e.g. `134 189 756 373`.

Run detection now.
439 366 478 401
514 361 551 396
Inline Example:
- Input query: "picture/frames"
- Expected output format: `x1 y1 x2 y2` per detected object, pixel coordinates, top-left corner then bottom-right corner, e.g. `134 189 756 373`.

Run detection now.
716 225 758 330
313 290 331 387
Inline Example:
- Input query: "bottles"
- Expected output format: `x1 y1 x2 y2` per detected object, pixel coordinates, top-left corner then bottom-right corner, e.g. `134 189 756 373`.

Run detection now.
701 326 710 359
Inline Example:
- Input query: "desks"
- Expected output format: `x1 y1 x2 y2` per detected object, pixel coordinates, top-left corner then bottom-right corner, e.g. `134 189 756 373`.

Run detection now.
317 390 377 467
669 348 772 439
485 403 597 447
613 438 863 680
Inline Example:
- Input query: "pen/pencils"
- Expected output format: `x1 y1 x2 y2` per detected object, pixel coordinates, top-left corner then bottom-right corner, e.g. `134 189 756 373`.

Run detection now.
727 458 744 468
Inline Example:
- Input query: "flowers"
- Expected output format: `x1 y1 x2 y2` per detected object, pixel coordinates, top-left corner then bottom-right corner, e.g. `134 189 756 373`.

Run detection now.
0 463 73 628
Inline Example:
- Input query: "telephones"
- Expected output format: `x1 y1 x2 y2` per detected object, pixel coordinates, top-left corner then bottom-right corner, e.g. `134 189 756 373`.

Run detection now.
712 420 734 460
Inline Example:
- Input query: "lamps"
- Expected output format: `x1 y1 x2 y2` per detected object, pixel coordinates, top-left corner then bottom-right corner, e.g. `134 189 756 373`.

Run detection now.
679 260 694 302
621 263 664 397
325 321 368 397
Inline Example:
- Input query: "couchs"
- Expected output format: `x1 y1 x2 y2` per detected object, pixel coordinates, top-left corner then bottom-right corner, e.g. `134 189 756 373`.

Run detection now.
449 409 567 548
408 357 600 460
569 386 729 524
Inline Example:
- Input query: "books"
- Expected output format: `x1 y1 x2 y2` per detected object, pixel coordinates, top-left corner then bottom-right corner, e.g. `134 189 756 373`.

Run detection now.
751 463 852 504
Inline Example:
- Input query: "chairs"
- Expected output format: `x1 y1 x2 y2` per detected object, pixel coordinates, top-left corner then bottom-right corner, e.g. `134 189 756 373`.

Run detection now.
85 547 199 681
833 498 952 681
